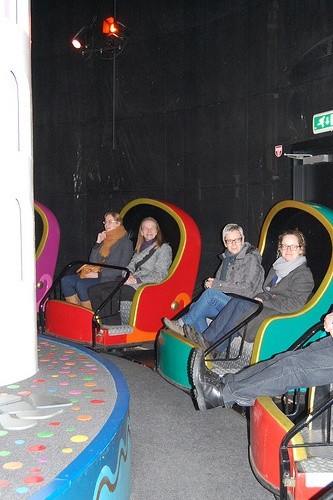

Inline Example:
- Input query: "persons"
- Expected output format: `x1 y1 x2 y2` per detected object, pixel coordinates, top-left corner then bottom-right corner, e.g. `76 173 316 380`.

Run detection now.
183 230 315 362
161 224 266 336
61 212 133 311
87 217 173 325
189 312 333 411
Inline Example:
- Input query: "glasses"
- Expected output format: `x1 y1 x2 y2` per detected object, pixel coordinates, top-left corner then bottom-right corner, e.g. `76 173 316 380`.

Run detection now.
281 245 302 251
103 221 118 224
225 238 241 244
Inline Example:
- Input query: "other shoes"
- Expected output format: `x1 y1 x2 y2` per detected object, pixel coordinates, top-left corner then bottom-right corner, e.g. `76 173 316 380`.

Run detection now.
183 324 214 354
162 318 186 336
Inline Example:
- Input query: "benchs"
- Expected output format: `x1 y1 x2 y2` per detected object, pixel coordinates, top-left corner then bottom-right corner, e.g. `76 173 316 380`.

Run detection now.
119 300 254 377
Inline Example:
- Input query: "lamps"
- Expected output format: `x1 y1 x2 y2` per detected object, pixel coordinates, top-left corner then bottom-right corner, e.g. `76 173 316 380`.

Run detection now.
72 15 128 60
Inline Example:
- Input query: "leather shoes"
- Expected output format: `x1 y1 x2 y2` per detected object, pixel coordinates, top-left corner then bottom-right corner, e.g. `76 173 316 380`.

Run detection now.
187 347 225 411
191 349 223 411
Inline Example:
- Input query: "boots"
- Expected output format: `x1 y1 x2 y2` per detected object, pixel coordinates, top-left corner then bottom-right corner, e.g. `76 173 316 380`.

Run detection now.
65 294 82 306
82 301 93 311
